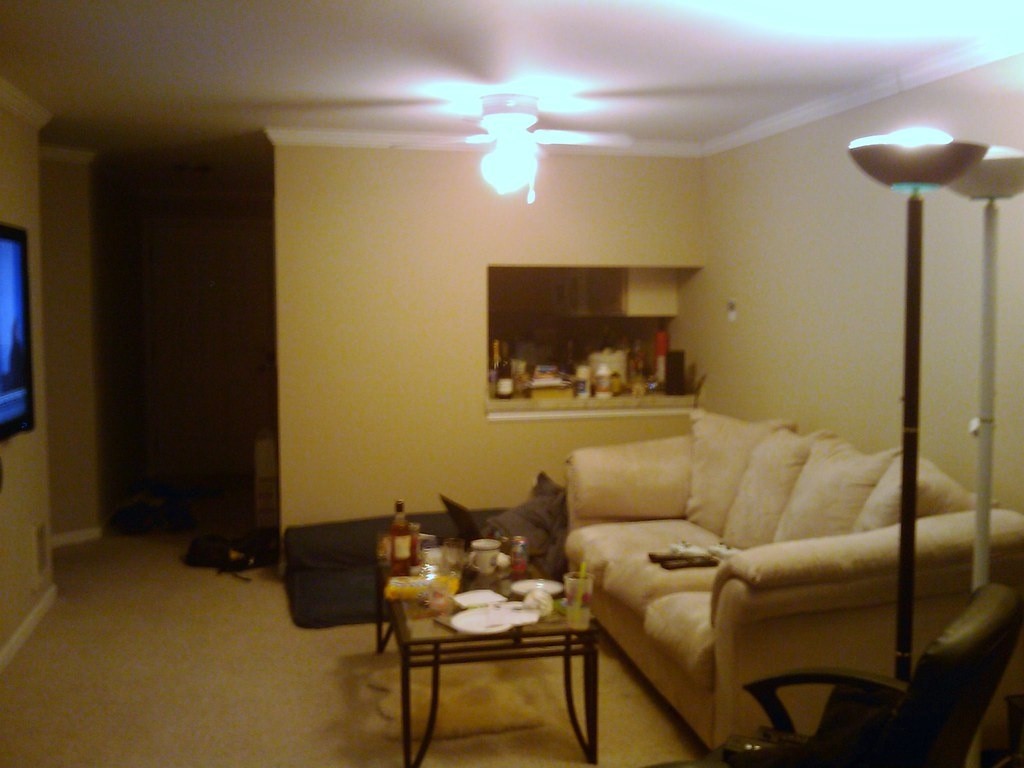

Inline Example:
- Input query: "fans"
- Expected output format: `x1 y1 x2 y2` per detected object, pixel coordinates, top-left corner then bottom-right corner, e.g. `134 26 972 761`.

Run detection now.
435 112 628 146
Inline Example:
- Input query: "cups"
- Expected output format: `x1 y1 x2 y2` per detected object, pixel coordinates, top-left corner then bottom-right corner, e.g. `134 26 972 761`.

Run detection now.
563 572 594 629
468 538 499 578
378 523 464 613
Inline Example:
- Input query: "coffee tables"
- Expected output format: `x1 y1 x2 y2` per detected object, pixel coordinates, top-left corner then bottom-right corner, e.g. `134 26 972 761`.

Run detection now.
375 569 601 768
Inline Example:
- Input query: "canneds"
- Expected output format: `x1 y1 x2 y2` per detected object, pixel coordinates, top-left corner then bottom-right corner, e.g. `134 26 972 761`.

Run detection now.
576 366 591 398
512 535 527 571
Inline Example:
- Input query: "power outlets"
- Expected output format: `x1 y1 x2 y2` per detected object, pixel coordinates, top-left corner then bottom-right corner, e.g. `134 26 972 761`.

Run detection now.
35 523 49 572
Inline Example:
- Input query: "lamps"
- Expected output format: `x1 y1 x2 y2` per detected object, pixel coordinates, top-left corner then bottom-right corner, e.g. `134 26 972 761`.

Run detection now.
926 149 1023 594
840 128 985 673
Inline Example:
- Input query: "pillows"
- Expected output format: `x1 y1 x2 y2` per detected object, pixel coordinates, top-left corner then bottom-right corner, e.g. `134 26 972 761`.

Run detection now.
771 438 903 544
719 428 836 552
683 407 796 533
848 450 1001 532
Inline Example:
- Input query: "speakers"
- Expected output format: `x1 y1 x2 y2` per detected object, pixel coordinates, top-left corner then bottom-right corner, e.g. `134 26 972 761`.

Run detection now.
665 350 685 395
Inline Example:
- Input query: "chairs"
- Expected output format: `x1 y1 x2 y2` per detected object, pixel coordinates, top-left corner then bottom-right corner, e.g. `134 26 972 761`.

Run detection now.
698 582 1024 768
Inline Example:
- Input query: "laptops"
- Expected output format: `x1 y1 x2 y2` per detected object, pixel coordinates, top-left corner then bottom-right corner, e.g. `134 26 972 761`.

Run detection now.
438 494 546 557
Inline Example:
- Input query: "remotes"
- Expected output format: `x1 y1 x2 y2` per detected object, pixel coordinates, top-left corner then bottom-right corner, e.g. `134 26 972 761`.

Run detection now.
649 551 719 570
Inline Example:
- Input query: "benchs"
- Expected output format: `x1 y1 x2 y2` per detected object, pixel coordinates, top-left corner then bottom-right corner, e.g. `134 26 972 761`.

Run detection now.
285 506 509 631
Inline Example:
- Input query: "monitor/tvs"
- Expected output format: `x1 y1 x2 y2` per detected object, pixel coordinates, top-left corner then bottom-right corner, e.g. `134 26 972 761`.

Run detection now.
0 222 35 441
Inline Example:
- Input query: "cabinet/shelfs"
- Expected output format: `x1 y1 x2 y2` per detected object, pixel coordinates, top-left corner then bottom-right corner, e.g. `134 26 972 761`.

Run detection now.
534 269 682 318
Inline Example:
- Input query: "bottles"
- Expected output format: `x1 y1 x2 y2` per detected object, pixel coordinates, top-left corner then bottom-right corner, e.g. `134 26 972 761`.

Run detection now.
390 500 411 576
524 581 554 618
489 325 684 399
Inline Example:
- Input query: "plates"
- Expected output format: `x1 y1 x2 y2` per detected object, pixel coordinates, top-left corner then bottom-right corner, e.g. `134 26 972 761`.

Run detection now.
510 578 564 595
451 602 515 634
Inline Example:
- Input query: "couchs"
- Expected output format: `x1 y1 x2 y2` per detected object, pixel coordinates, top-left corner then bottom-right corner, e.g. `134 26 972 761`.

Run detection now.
561 404 1024 746
